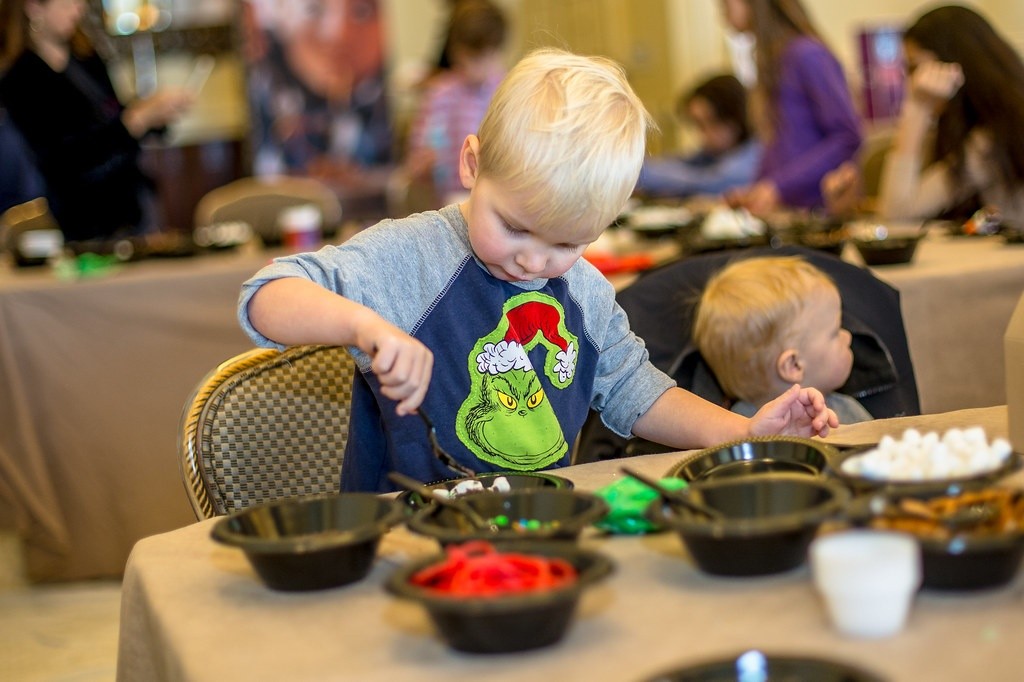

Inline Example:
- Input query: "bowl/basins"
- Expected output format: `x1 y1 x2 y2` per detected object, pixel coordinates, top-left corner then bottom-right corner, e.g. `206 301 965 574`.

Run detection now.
609 211 926 266
394 471 574 515
408 490 609 551
210 493 409 592
646 470 850 581
385 543 612 655
661 435 840 479
830 443 1024 593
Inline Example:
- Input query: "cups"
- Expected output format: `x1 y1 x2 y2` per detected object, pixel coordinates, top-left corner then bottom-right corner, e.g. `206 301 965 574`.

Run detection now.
807 527 922 638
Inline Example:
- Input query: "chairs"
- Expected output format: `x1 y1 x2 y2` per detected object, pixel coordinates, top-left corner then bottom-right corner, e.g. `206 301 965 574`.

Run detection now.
577 246 920 466
179 346 356 523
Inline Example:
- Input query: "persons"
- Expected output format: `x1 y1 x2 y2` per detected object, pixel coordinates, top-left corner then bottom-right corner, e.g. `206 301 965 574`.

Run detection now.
634 73 765 200
245 0 390 233
677 246 873 424
682 0 862 214
0 0 196 266
401 0 516 206
823 4 1024 235
238 19 838 494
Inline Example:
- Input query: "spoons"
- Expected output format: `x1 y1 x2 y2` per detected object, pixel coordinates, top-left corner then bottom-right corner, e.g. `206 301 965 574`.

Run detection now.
419 405 477 477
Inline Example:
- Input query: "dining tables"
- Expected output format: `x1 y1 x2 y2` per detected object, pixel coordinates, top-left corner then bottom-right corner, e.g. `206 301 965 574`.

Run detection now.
0 178 1024 682
